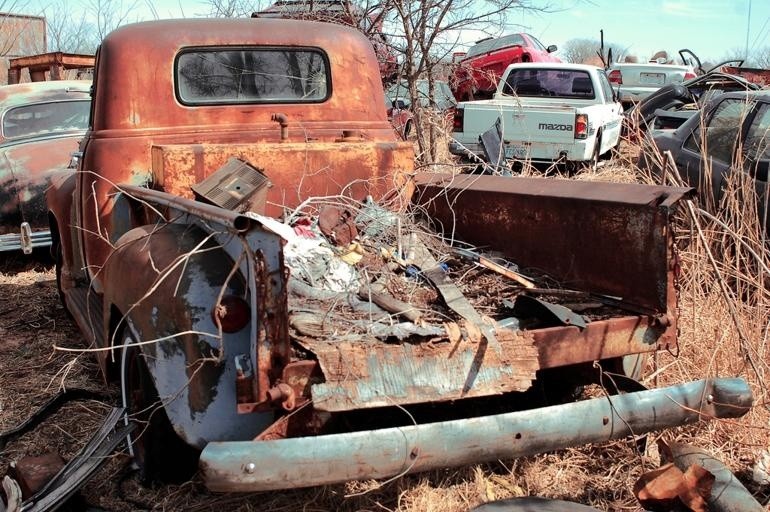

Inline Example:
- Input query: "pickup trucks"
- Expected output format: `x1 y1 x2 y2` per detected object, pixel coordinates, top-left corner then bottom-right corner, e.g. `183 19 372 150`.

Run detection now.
52 23 680 476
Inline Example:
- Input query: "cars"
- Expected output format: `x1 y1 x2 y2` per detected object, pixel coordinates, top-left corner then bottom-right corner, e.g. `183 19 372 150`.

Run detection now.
638 89 770 233
608 51 697 104
389 32 623 171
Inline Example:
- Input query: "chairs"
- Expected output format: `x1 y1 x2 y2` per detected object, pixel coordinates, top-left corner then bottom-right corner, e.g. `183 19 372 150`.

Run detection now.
624 55 638 63
515 79 541 92
571 76 593 97
